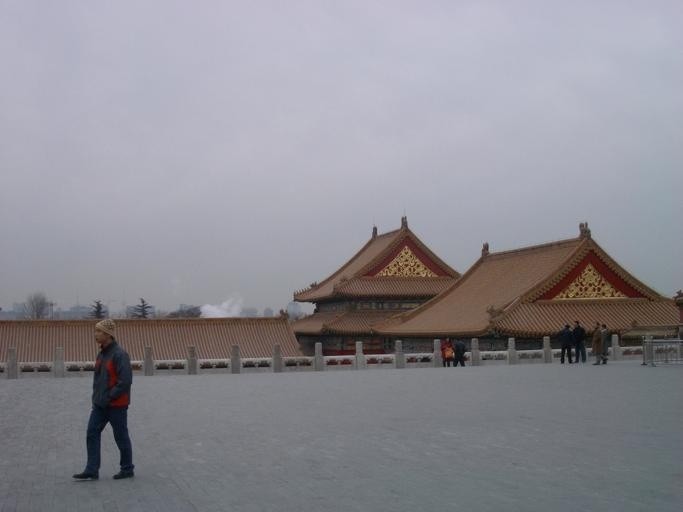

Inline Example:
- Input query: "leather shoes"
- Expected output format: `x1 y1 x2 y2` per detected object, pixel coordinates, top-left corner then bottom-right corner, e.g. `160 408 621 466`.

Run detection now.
72 472 99 479
113 470 133 479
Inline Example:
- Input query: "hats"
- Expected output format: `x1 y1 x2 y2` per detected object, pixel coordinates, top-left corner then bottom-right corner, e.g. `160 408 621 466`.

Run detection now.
95 319 116 338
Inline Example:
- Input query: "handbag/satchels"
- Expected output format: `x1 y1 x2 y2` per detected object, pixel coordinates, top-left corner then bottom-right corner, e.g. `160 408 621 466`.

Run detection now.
446 350 453 358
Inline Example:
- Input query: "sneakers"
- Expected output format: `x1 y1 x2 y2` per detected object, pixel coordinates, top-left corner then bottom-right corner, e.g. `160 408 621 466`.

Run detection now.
592 361 606 365
561 358 585 364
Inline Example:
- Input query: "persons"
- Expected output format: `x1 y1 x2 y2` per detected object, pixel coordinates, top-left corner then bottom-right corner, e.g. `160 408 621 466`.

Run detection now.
440 335 452 368
572 320 587 363
452 337 465 367
558 324 573 364
70 317 134 478
599 324 610 364
589 321 602 366
